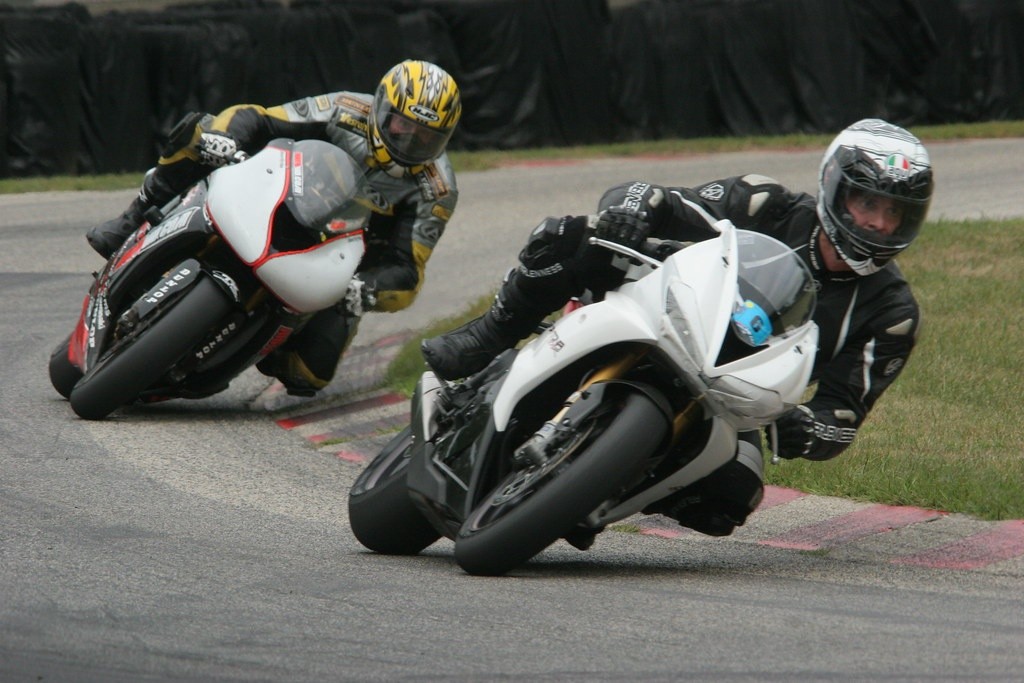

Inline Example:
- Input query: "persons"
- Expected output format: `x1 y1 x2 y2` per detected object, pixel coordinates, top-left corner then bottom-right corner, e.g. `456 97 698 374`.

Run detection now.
422 118 935 550
85 58 459 398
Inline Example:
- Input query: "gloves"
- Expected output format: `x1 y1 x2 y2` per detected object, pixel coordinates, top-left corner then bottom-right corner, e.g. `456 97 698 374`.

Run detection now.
341 278 375 321
597 203 647 259
764 405 815 460
201 131 238 170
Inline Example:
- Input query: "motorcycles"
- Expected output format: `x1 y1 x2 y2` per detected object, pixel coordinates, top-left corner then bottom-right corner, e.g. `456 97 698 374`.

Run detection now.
47 136 375 421
348 218 821 576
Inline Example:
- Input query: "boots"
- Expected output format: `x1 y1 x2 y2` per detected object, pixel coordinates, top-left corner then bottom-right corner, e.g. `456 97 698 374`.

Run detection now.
421 270 544 381
86 165 178 258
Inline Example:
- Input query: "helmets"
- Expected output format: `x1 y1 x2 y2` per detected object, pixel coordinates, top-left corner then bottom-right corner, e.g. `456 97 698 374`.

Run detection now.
366 59 461 180
817 116 935 278
